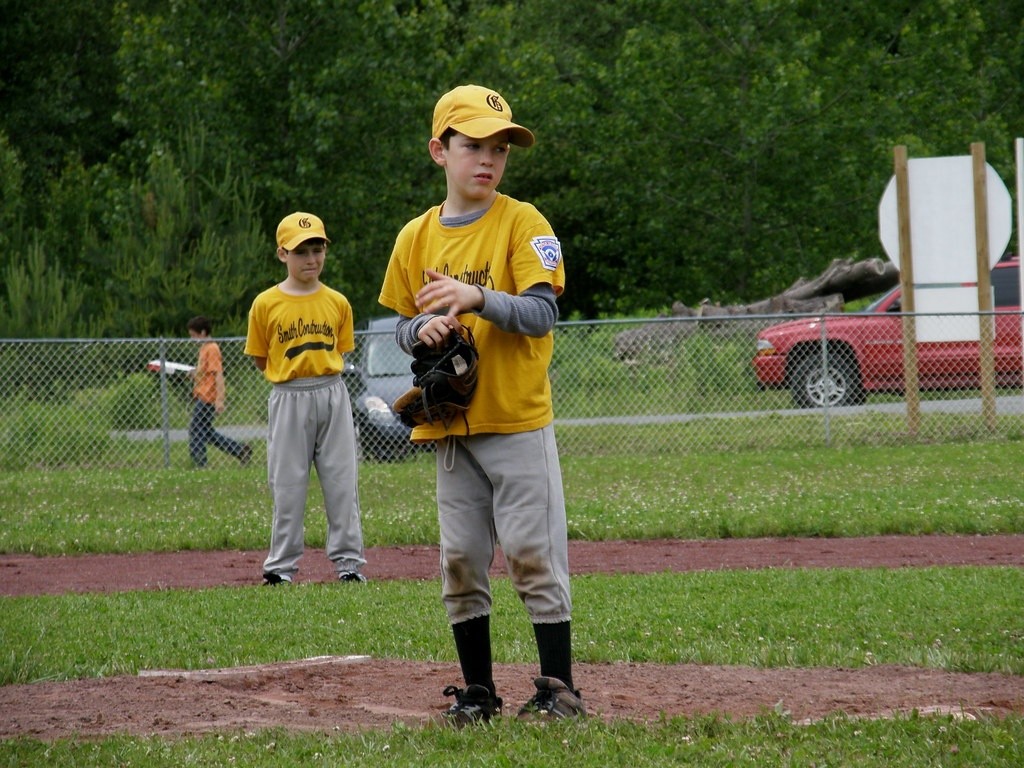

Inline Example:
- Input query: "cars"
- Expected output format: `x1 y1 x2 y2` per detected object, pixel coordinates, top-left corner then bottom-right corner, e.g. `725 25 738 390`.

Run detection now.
751 261 1024 408
340 317 437 461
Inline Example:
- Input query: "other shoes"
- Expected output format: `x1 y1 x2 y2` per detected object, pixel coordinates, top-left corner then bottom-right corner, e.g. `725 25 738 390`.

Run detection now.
263 572 292 587
240 444 253 469
338 570 367 585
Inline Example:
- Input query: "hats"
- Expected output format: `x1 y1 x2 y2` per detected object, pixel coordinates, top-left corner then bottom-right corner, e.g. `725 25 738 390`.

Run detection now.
432 85 534 148
276 212 331 251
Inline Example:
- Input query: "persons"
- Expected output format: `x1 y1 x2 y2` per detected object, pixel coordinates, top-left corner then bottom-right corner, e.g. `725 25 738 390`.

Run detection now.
377 84 587 728
245 211 367 585
188 316 254 472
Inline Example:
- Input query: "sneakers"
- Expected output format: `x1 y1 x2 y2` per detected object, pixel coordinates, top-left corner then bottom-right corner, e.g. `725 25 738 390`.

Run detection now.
443 684 503 730
514 677 586 722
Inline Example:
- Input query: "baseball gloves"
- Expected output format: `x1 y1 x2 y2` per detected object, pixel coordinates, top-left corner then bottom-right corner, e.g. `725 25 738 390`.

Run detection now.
407 325 483 431
